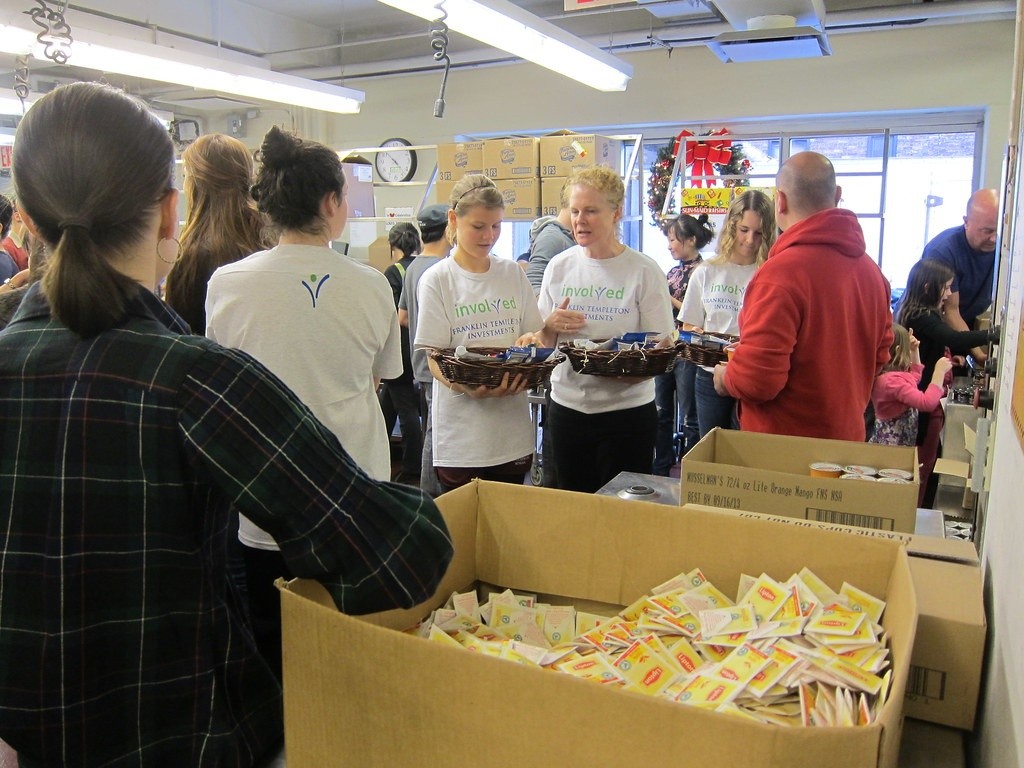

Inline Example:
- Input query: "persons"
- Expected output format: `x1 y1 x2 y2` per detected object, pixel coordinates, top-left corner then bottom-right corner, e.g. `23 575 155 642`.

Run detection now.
0 83 454 768
383 220 423 485
867 322 953 445
894 188 999 367
514 165 679 490
713 151 891 443
398 203 454 498
201 124 405 768
896 258 1001 507
166 129 277 337
0 192 48 329
655 213 714 478
676 189 776 446
414 173 547 492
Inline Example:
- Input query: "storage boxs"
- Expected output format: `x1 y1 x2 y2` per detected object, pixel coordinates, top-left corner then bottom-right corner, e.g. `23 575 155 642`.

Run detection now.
276 129 996 768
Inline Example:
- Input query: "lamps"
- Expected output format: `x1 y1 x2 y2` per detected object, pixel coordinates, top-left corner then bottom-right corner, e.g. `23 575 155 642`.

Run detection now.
0 8 366 114
377 0 633 91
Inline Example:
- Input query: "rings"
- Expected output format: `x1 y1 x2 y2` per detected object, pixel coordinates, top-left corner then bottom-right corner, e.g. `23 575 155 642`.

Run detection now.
564 322 567 330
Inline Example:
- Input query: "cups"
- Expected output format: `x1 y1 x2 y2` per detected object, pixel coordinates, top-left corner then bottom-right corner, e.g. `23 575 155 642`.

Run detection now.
878 469 913 480
877 477 910 482
719 360 728 365
727 348 736 362
842 465 877 477
838 474 878 484
953 382 985 405
810 463 842 479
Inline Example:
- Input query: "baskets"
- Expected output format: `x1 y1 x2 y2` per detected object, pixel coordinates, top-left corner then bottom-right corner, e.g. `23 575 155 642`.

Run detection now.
560 339 687 377
431 346 566 387
681 332 741 367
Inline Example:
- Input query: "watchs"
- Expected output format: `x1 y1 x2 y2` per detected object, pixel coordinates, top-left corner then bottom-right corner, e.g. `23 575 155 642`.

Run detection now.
3 277 14 289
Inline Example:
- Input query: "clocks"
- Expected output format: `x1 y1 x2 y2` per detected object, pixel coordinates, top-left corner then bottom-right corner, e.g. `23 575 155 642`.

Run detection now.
375 137 417 182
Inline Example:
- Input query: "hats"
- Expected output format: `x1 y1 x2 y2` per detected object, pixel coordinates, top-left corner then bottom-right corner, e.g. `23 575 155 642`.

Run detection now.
417 204 453 226
